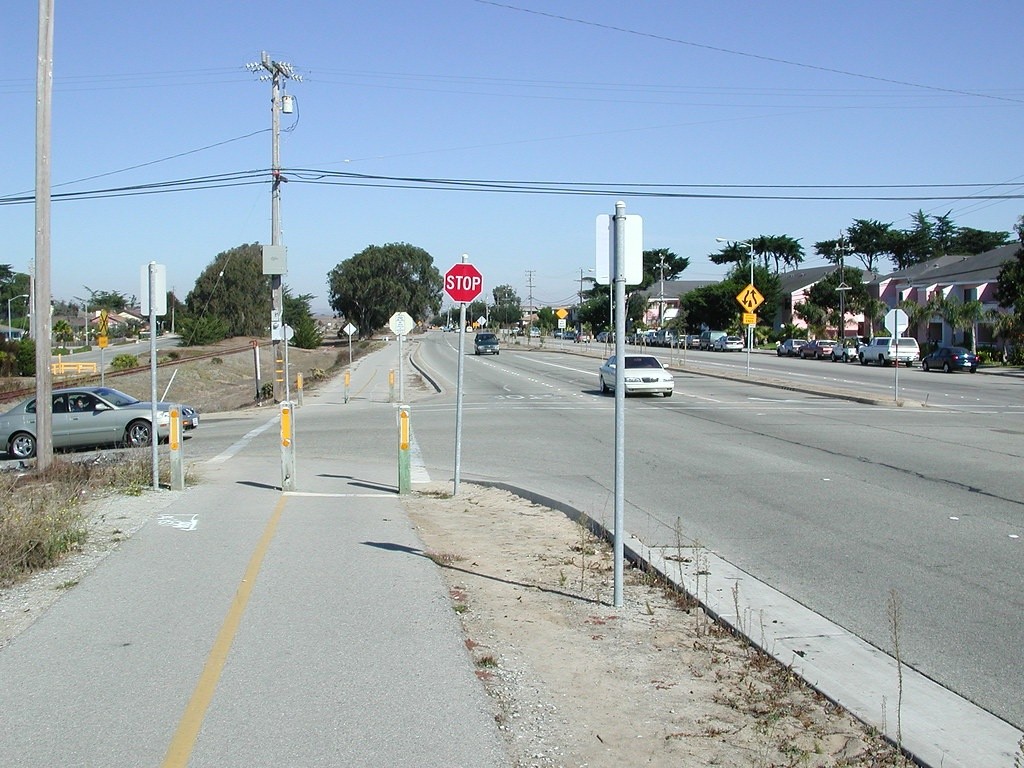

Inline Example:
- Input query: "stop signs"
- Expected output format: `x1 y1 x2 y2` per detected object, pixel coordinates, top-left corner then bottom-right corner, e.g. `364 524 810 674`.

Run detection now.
445 263 482 303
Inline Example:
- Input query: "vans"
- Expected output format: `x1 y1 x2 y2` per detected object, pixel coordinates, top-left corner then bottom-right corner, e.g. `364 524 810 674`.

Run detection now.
698 331 727 351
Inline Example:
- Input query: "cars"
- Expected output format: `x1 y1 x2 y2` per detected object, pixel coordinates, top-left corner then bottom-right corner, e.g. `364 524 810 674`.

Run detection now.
777 338 809 356
512 327 523 335
554 329 702 350
1 384 200 459
598 354 675 397
529 327 542 338
712 335 744 351
443 327 449 333
466 326 471 333
474 331 500 356
454 329 460 332
921 346 980 375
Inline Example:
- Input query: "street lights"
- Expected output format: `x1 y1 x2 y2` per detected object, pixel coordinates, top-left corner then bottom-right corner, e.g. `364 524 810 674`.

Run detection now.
715 237 755 286
7 293 28 343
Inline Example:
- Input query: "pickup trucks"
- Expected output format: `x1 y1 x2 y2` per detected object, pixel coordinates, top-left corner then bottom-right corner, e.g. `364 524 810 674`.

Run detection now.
830 338 864 364
799 339 837 360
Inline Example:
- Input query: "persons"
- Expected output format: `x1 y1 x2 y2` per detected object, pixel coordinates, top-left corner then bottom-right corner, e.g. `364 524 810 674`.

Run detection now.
71 396 87 412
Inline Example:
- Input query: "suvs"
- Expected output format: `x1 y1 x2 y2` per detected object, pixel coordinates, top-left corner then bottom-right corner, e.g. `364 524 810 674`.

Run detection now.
858 336 921 368
